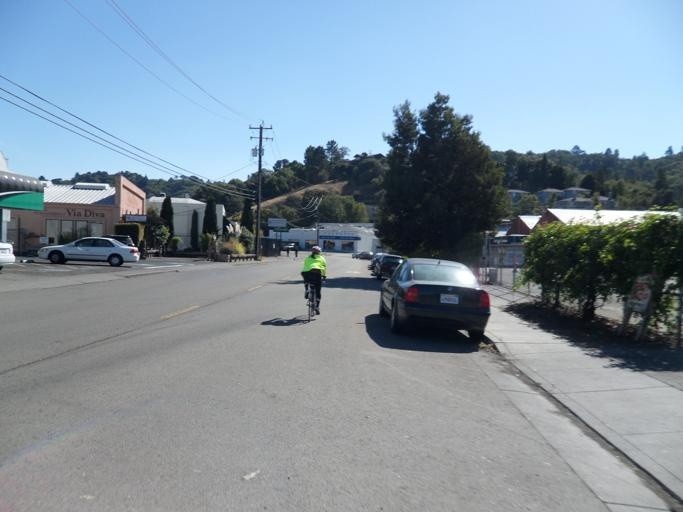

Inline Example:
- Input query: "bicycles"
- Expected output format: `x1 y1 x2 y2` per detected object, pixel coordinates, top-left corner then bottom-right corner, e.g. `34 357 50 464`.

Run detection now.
303 274 327 322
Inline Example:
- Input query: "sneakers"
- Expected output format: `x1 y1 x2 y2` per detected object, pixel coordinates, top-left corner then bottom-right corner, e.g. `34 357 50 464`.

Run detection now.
315 308 320 315
305 291 309 299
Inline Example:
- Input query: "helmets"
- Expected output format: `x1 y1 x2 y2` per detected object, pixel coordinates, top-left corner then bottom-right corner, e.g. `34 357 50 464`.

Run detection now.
310 246 321 253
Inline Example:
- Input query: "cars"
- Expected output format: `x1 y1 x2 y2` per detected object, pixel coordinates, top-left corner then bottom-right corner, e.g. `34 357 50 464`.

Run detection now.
283 242 296 251
37 234 140 267
352 250 491 344
1 241 16 272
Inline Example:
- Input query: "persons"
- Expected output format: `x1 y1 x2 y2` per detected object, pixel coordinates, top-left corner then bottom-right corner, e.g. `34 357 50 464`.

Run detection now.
301 245 329 316
